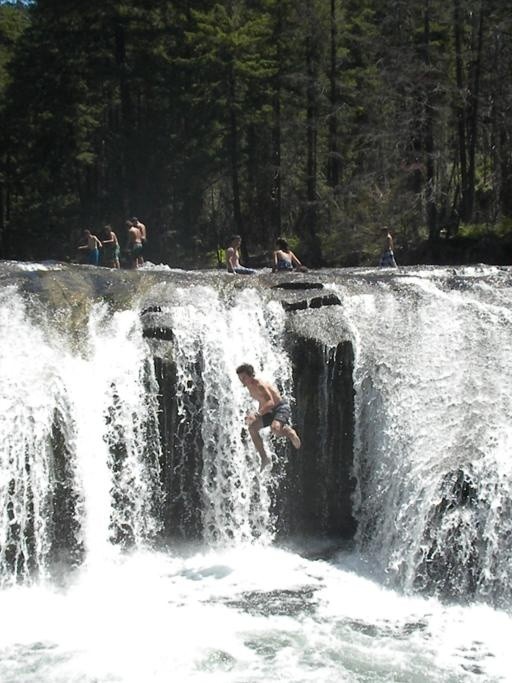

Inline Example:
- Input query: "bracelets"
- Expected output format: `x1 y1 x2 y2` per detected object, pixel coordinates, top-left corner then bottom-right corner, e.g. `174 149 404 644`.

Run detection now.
254 411 262 418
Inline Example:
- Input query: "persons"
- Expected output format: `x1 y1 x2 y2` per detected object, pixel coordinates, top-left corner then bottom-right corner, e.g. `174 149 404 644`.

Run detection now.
125 220 144 268
271 238 301 273
379 226 397 268
77 229 103 266
132 217 147 244
101 224 121 269
236 364 301 472
226 234 255 275
